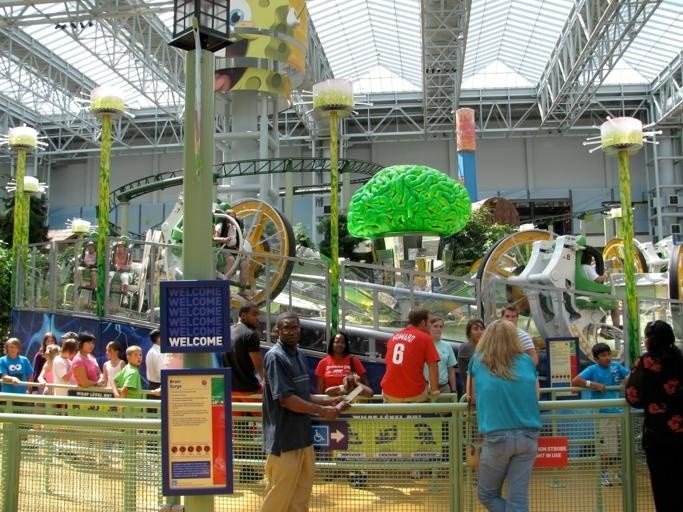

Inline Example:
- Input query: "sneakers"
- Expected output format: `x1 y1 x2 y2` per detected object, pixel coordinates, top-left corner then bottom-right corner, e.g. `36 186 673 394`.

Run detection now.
601 473 613 486
616 471 624 483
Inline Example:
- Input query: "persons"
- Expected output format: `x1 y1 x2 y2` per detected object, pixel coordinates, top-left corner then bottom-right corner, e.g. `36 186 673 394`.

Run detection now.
73 241 99 293
582 255 623 339
213 209 249 296
109 241 132 299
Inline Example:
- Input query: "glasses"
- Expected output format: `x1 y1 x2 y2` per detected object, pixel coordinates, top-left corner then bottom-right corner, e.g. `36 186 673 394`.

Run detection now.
282 326 300 333
